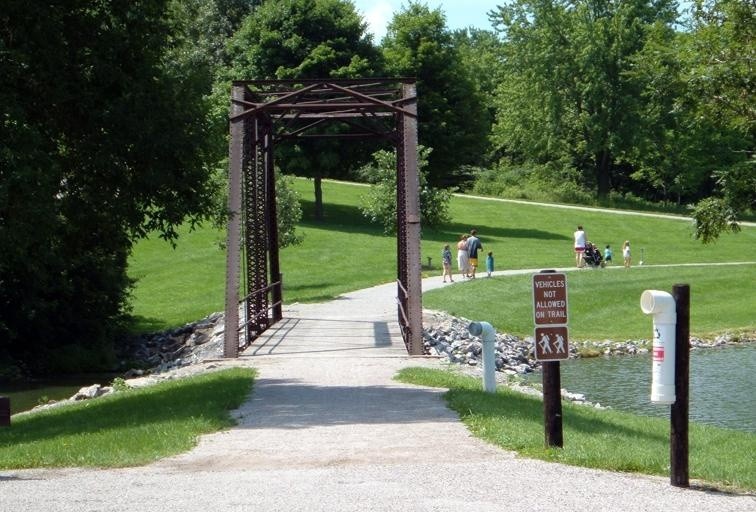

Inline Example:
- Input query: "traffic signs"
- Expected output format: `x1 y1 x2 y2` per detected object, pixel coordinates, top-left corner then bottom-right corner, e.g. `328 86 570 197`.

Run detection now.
531 272 567 327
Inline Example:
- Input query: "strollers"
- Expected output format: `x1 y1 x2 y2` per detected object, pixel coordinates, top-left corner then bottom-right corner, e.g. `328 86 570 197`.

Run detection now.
582 239 605 269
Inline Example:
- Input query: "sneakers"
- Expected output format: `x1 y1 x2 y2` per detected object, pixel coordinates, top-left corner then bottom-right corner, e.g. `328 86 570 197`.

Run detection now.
463 275 475 279
444 280 454 283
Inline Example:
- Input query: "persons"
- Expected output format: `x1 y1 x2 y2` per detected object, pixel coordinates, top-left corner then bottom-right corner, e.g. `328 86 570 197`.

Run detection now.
456 233 470 278
465 230 485 280
574 224 589 268
441 244 455 283
485 250 495 278
605 244 614 265
623 241 633 267
